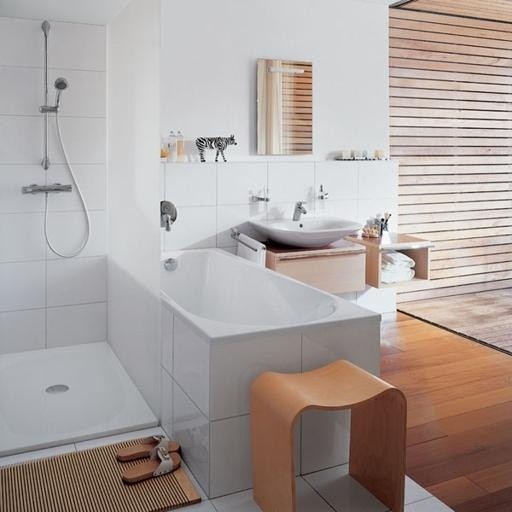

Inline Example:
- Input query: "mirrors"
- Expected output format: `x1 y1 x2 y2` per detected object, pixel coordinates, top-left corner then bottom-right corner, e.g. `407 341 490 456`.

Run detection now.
257 58 314 157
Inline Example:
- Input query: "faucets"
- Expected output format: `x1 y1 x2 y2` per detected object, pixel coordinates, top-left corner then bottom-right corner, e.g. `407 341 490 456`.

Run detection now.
293 201 307 221
163 214 172 231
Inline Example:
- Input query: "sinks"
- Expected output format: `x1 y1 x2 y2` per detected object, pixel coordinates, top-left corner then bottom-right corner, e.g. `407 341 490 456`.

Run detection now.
248 215 362 247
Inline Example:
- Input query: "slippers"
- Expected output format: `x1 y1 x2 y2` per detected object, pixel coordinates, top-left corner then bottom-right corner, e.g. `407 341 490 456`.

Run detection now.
115 432 180 462
120 451 180 484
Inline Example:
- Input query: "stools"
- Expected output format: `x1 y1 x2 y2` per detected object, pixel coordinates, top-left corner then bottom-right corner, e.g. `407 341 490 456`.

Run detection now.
249 359 408 512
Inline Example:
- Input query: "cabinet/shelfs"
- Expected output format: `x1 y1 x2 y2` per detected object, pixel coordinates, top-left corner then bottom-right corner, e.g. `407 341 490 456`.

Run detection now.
344 234 435 289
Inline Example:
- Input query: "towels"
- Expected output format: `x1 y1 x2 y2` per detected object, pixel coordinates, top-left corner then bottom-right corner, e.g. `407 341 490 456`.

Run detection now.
237 232 267 267
380 252 415 281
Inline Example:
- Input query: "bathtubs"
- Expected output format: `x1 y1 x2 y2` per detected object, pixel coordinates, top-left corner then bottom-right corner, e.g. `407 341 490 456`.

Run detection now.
107 247 381 500
